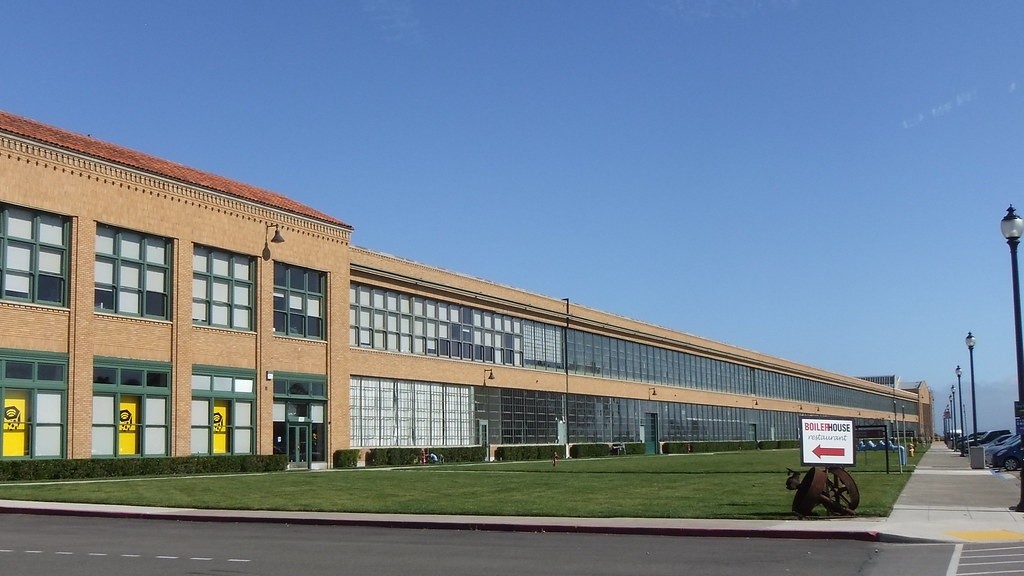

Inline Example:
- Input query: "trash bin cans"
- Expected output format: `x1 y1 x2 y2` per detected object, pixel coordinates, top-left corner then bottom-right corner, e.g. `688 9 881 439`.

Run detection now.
970 446 986 469
948 440 953 449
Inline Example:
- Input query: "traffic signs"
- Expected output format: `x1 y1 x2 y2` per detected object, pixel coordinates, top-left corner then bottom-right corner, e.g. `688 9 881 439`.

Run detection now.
799 413 856 467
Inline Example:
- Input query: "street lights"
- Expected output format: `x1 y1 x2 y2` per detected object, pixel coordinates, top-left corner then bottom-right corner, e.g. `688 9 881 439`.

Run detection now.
956 365 966 457
965 332 978 446
943 394 954 450
951 385 958 452
1000 204 1024 513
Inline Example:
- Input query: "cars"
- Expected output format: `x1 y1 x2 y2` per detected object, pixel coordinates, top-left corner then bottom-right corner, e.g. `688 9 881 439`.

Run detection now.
954 430 1024 471
